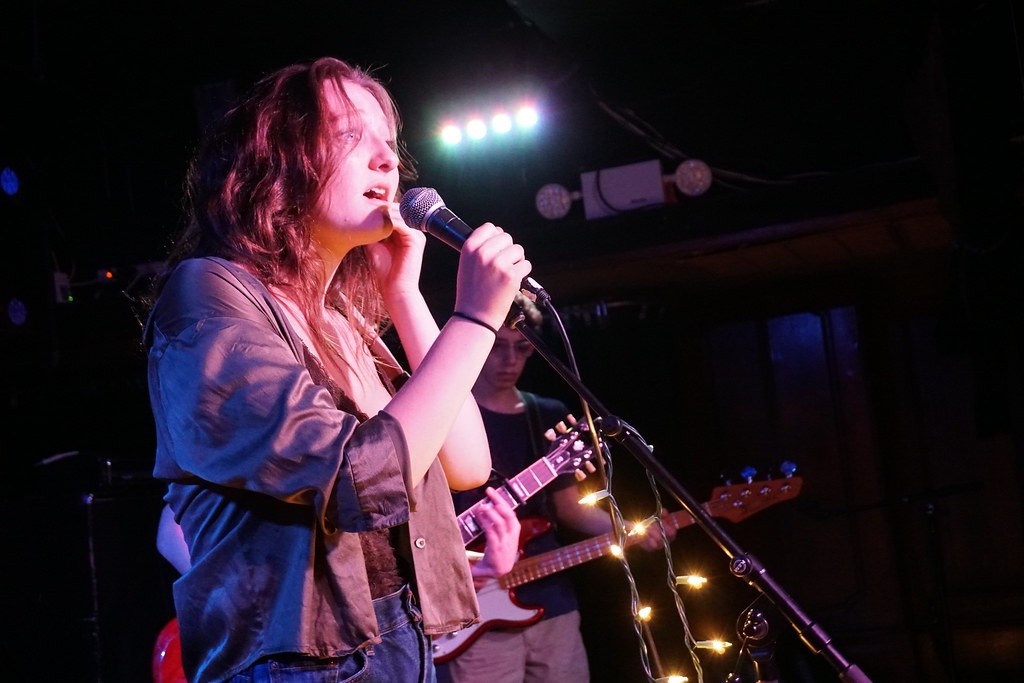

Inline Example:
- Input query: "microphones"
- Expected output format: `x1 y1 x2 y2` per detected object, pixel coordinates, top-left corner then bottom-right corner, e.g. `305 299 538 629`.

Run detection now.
398 187 552 308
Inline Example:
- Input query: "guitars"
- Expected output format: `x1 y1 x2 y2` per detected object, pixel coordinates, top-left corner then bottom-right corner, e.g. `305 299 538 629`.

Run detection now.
425 460 807 666
151 410 612 682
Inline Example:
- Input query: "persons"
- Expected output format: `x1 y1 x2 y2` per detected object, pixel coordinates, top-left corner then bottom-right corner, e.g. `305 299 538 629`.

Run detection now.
448 288 677 683
138 56 532 683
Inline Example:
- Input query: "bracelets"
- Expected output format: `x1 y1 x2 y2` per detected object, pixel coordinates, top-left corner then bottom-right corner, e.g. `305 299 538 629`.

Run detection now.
453 311 499 338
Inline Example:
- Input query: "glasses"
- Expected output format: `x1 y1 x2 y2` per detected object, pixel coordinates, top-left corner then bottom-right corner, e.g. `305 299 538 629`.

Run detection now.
489 341 534 357
